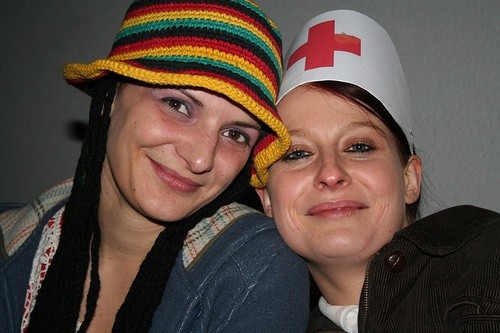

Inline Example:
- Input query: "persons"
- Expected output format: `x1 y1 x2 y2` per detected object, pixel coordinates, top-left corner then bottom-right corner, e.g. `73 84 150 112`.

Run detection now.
0 0 310 333
254 9 500 333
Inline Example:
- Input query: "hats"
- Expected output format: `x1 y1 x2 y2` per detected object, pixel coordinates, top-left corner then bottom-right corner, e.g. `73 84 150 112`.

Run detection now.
61 0 291 188
274 9 414 155
